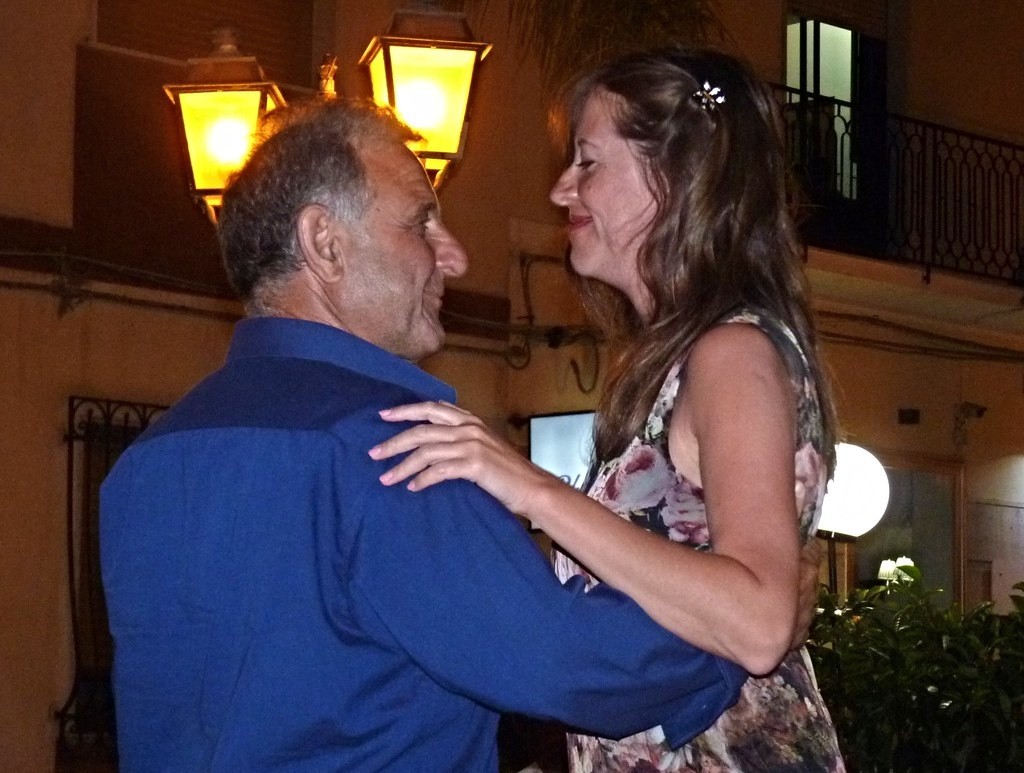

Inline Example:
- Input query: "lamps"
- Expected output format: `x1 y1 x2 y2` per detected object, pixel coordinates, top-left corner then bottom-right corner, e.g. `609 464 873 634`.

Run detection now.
65 1 496 274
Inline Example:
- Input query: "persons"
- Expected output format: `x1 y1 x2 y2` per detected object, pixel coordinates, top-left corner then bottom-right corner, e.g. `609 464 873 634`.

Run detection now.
101 100 826 773
368 39 848 773
